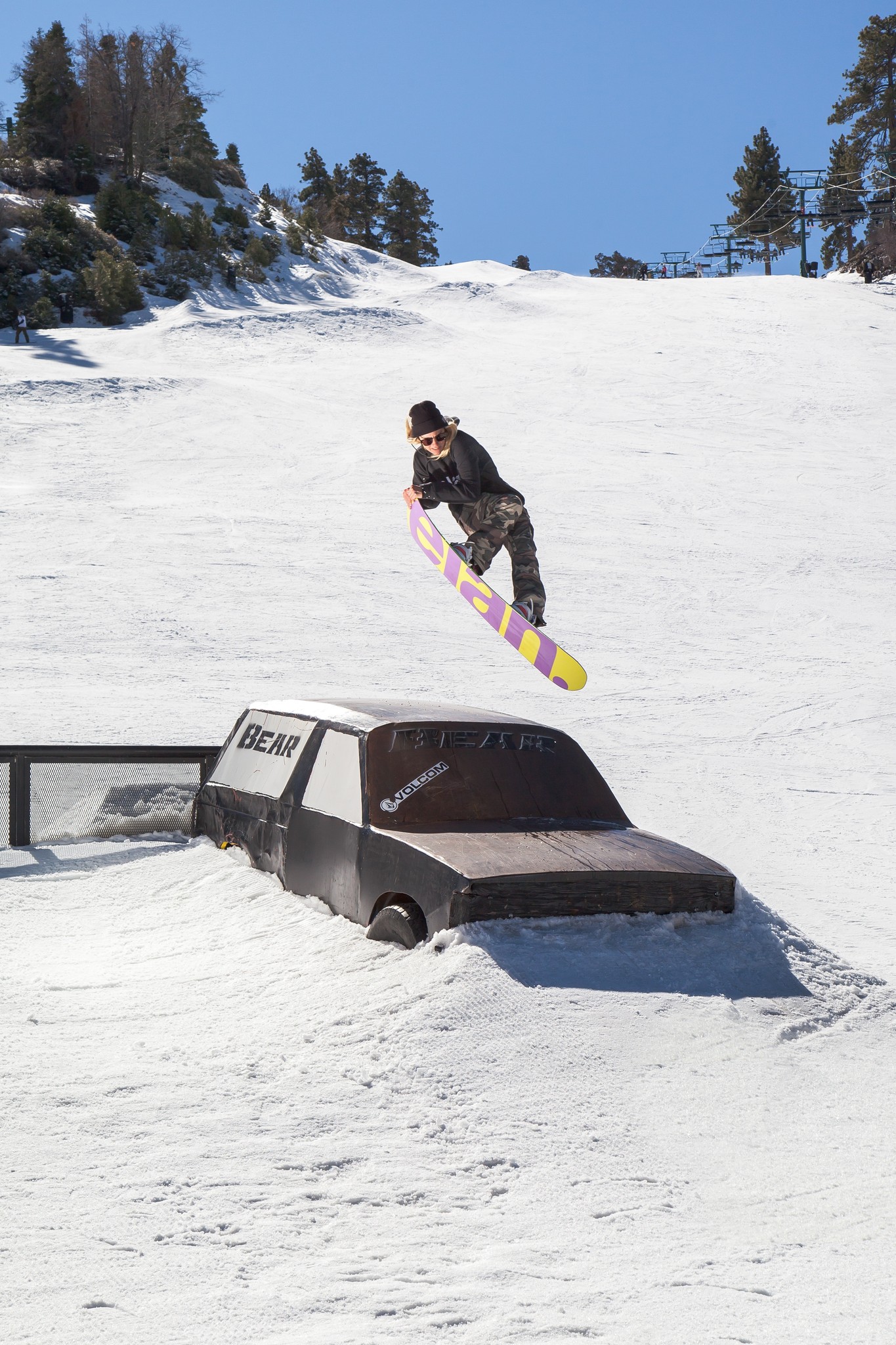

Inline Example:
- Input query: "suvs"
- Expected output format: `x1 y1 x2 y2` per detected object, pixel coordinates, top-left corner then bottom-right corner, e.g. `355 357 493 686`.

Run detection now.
189 692 741 948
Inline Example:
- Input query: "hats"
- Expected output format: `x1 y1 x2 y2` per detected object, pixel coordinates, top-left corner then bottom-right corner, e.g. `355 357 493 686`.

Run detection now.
407 400 450 439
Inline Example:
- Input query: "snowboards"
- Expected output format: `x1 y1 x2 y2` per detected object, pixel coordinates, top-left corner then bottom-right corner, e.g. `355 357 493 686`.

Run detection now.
406 489 587 691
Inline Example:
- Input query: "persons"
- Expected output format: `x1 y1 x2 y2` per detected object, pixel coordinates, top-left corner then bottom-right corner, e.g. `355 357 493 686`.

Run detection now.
401 398 550 629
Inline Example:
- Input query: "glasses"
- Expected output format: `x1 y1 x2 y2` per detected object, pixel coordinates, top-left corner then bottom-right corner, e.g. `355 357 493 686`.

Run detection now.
414 428 450 446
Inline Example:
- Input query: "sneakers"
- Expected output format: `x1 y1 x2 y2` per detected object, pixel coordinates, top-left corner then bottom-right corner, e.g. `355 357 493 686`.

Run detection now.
507 597 539 627
449 539 480 576
